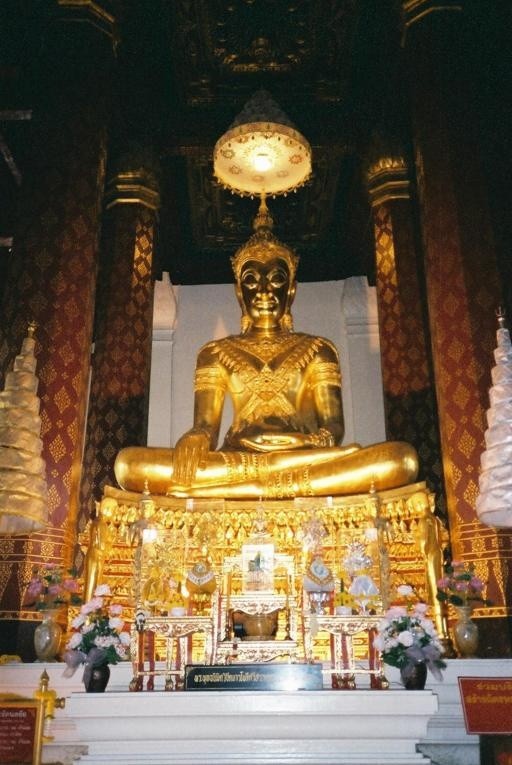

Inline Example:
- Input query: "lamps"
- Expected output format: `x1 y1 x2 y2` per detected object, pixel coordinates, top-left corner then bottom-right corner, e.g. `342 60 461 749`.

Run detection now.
206 80 316 203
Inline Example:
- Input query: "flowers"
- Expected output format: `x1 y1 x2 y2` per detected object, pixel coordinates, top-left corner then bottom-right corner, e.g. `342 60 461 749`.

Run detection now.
435 558 493 608
24 561 84 610
66 583 131 663
372 585 446 682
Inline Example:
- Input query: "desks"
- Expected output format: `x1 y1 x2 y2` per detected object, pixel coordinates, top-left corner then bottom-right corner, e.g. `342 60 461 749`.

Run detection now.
129 616 208 692
304 614 390 692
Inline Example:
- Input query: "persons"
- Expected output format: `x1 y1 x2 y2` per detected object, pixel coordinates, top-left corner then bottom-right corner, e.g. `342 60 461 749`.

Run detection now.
110 225 422 496
248 506 272 539
128 479 166 549
363 497 386 534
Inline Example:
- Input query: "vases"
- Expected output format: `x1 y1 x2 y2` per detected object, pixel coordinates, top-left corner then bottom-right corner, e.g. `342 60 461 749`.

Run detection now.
450 601 481 659
401 652 429 690
83 649 111 693
33 608 63 661
